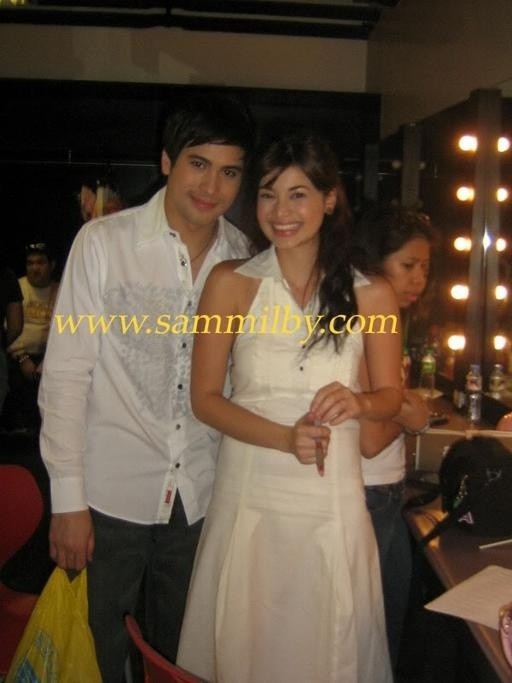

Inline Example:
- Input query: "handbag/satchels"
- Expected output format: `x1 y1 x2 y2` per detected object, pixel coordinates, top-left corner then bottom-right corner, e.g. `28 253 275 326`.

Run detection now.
441 436 512 537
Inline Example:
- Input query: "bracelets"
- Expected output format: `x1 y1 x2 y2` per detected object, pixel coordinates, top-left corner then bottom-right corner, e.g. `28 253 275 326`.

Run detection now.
405 420 430 437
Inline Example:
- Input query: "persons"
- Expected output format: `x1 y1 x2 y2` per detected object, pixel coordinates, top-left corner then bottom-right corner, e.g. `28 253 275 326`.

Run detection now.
35 98 259 683
360 207 430 678
0 178 62 428
175 137 403 683
80 170 126 221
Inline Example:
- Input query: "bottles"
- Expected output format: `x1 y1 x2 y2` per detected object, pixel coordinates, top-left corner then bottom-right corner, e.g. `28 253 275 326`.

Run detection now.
420 325 441 374
400 346 412 400
464 364 484 425
419 349 436 405
488 364 509 395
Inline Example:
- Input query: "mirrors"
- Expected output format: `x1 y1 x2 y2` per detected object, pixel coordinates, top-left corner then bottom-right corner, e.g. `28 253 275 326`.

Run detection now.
380 82 511 425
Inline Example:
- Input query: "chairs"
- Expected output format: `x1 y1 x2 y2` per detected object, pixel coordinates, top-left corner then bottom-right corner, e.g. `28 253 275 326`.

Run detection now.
124 615 208 682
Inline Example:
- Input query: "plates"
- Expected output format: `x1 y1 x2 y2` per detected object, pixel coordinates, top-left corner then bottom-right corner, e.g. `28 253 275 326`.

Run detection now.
407 388 443 401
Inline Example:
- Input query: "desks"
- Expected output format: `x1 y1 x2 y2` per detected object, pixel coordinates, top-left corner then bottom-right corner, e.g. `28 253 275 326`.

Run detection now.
400 384 512 683
